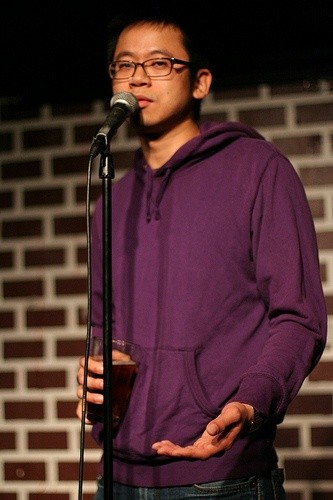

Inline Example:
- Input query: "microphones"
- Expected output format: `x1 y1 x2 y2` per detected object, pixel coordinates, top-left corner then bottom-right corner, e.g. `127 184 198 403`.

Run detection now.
89 92 136 157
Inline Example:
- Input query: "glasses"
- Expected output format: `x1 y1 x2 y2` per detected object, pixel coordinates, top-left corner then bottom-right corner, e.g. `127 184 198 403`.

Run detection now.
107 57 191 80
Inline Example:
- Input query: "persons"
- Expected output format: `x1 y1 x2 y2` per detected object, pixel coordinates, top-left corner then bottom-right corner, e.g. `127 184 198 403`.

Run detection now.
75 8 329 500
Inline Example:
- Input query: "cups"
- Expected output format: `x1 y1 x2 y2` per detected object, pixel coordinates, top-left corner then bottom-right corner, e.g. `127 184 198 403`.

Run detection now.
83 334 145 423
